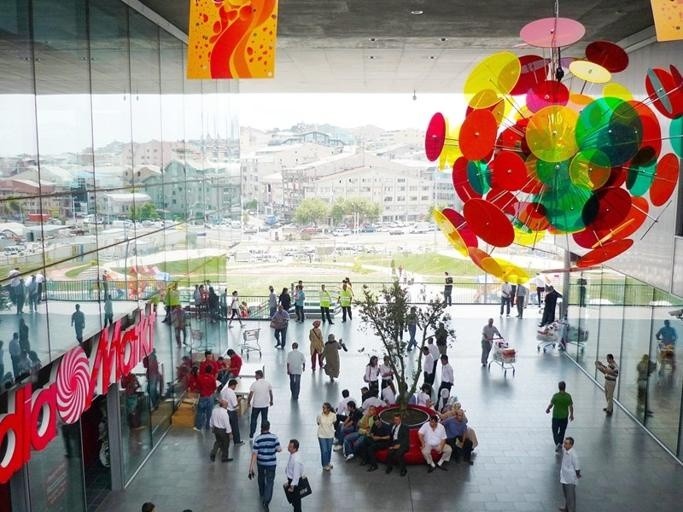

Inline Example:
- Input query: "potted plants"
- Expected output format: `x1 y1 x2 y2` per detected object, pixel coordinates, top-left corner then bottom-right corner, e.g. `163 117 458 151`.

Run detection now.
355 279 457 426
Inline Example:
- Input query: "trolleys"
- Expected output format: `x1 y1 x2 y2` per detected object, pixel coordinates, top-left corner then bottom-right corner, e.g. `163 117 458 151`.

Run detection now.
566 325 589 356
536 321 565 353
187 325 216 354
240 327 262 359
656 338 676 375
487 337 515 377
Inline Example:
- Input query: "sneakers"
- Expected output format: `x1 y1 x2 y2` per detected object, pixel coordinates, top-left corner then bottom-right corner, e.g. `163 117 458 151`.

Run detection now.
333 437 354 462
275 343 284 349
500 314 523 318
555 443 562 452
192 427 200 432
328 464 333 468
295 317 353 324
323 465 330 471
228 324 248 328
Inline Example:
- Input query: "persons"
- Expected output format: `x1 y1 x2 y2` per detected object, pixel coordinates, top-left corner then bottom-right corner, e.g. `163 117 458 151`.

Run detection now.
595 353 619 416
247 420 282 512
637 354 657 413
319 334 343 382
247 369 274 438
435 322 448 355
444 271 454 307
142 502 155 512
391 263 416 286
227 276 357 328
284 439 305 512
119 346 164 434
334 337 479 477
546 380 575 454
308 320 325 370
316 402 337 471
577 272 588 307
405 307 424 352
286 341 306 400
1 264 114 392
271 305 289 350
559 436 580 512
656 319 678 370
150 280 225 350
481 317 503 367
500 280 562 327
189 348 245 463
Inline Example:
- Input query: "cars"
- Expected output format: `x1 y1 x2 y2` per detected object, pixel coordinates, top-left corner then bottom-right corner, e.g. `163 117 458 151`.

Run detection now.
590 298 610 304
301 221 438 235
272 223 280 228
286 223 297 227
235 249 281 264
284 247 381 257
231 221 241 228
194 229 206 236
126 219 159 229
260 226 269 232
649 300 670 305
50 219 60 224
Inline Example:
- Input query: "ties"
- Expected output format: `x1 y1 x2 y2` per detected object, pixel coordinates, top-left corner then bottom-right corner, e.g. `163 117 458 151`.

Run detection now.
392 425 398 439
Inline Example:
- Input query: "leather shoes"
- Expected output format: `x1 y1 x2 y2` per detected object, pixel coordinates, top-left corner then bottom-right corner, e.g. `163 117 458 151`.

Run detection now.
234 441 243 444
606 412 612 417
464 457 473 465
360 460 368 466
603 408 607 411
559 506 566 511
437 462 448 471
385 467 392 473
224 458 233 461
263 501 269 512
249 432 253 438
400 469 407 476
368 464 378 472
210 453 215 462
428 464 436 473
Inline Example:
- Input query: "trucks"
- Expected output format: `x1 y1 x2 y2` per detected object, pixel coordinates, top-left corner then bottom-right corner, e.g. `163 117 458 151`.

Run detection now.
265 215 278 225
243 228 257 234
160 220 175 232
0 231 6 240
82 214 103 224
25 214 49 223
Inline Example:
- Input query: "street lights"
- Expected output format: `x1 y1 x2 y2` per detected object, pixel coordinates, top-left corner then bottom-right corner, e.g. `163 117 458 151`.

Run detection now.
351 202 356 236
72 195 77 219
356 202 361 235
103 191 109 224
189 200 198 227
254 197 258 215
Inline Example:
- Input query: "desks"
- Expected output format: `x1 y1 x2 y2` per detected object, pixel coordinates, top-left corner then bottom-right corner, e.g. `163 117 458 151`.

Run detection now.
221 378 255 407
239 363 265 380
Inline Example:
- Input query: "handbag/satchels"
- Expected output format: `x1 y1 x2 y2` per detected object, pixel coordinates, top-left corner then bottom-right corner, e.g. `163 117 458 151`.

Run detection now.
364 374 370 382
283 476 312 503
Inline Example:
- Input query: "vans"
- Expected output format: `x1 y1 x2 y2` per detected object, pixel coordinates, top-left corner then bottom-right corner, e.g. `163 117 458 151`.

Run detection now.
3 245 18 256
